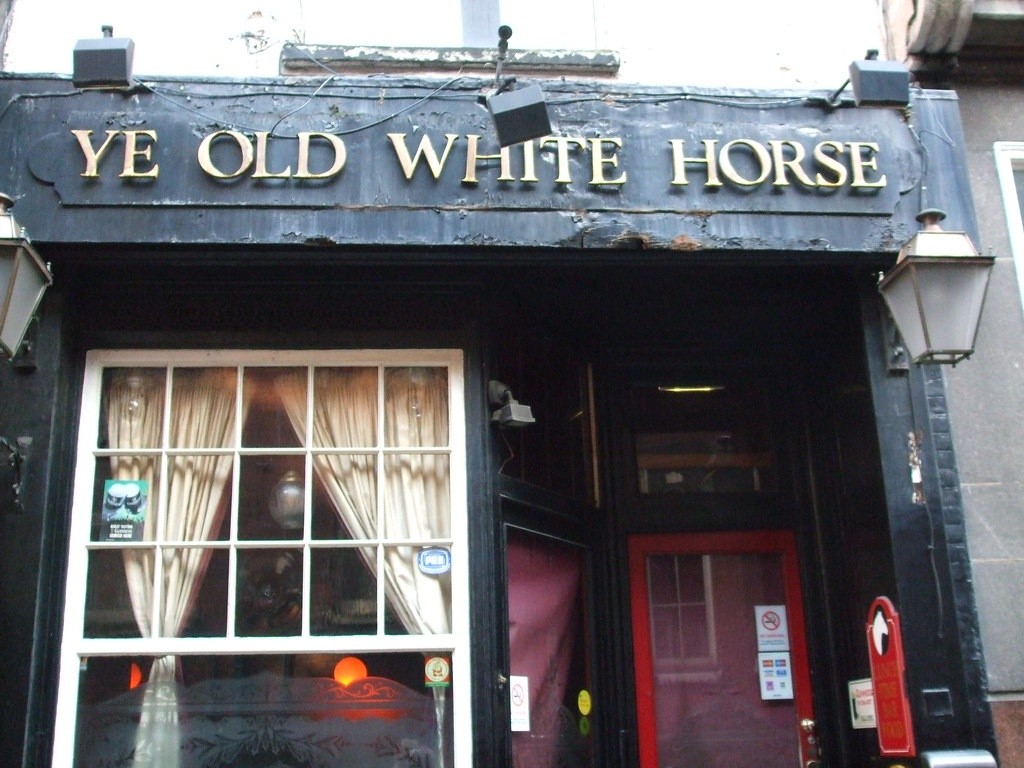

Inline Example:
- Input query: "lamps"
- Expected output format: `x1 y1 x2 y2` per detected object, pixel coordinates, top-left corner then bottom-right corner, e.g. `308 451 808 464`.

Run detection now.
269 466 313 529
476 23 554 148
826 48 912 112
0 187 54 371
72 22 138 99
489 378 536 429
871 208 996 376
